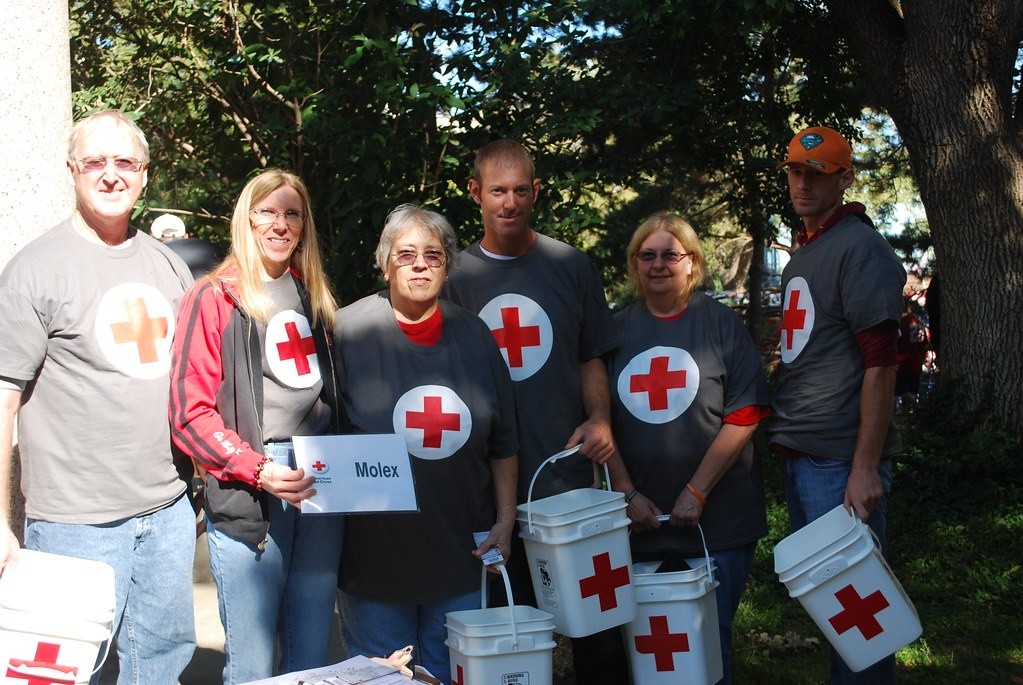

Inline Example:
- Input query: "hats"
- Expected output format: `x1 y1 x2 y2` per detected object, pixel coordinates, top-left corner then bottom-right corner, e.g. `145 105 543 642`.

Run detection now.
151 213 186 239
775 126 853 174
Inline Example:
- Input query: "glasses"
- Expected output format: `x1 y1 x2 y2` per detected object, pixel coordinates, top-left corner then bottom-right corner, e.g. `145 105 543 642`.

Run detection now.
389 250 448 268
634 249 694 262
251 208 306 221
80 156 144 172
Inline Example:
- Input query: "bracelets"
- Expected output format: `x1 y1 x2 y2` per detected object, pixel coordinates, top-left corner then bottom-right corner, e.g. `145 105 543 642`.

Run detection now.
687 484 706 506
253 456 273 492
625 489 638 502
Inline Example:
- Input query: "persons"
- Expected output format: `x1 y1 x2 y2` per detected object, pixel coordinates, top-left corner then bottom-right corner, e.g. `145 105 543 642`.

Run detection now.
438 141 629 685
0 109 205 685
334 204 518 685
151 213 224 281
605 213 771 685
168 167 342 685
770 127 907 685
